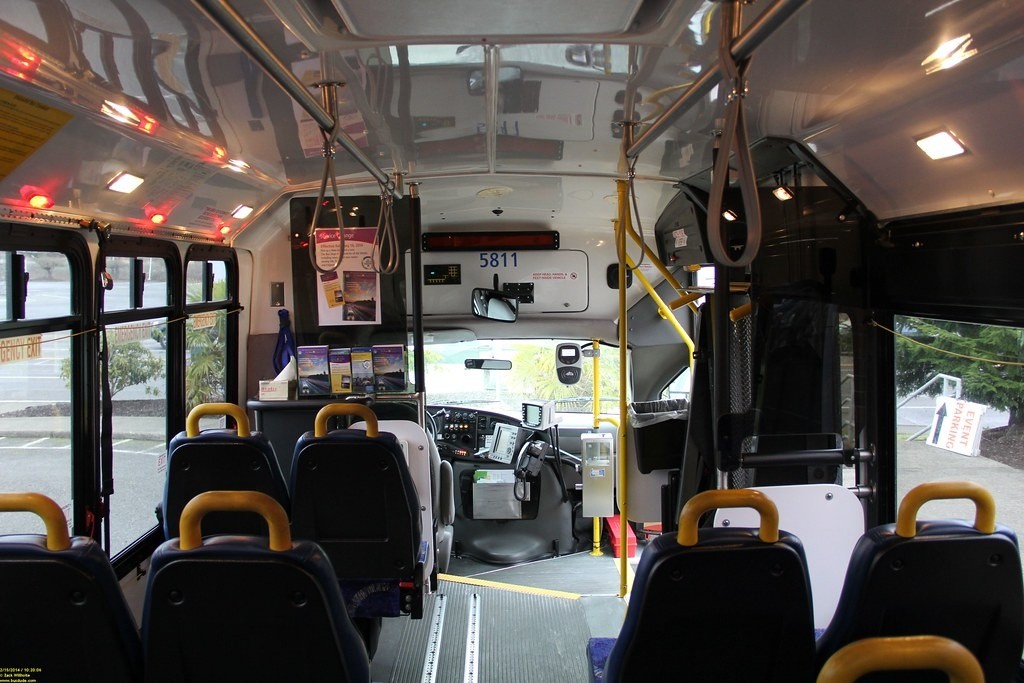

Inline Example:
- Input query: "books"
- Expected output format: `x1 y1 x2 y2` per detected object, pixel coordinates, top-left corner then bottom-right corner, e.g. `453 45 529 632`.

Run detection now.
297 344 405 395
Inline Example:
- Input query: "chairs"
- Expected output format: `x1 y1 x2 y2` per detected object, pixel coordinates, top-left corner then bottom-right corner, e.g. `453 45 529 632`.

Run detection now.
587 480 1024 683
0 402 426 683
